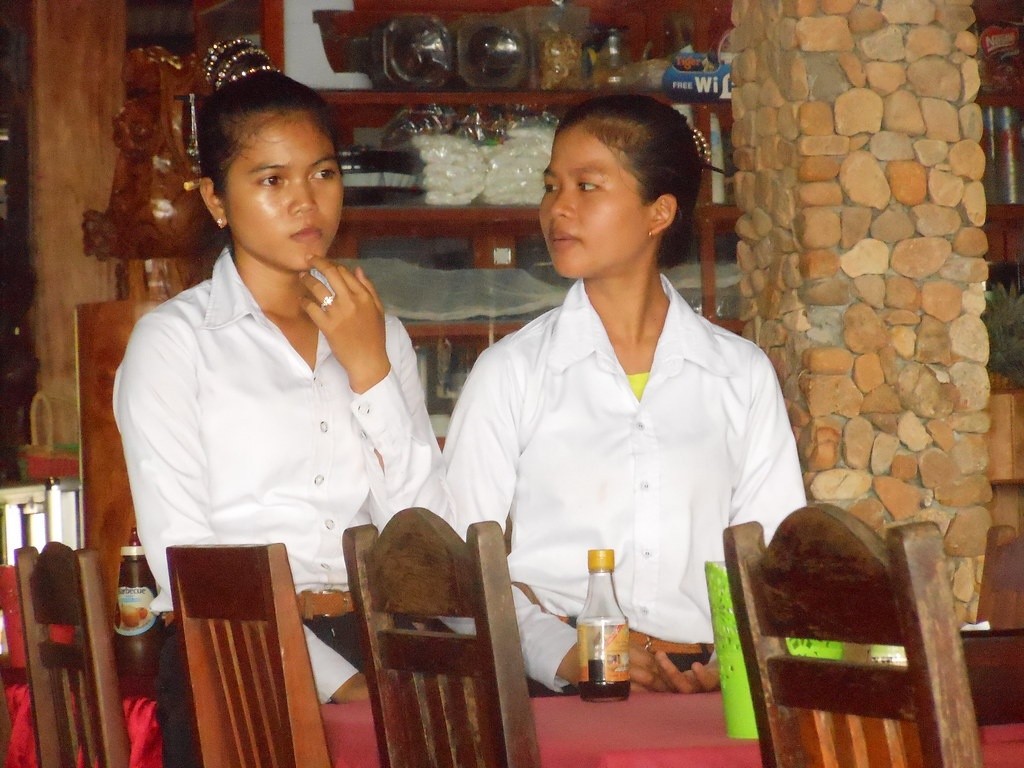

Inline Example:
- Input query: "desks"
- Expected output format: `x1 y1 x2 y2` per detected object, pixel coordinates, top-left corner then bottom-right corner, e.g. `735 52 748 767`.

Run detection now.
0 678 1024 768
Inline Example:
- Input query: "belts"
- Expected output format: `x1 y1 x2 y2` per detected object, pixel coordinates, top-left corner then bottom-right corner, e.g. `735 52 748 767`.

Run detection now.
166 589 355 624
515 580 715 655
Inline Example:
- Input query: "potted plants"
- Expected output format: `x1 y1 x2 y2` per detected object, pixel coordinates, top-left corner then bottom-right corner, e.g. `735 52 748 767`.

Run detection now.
982 285 1024 484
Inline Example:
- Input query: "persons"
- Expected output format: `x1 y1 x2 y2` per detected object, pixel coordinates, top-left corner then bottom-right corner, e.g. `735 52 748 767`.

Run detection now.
415 91 806 693
112 69 453 704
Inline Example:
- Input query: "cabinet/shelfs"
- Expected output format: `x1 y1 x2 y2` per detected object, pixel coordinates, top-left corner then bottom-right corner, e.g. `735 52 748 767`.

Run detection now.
311 0 745 453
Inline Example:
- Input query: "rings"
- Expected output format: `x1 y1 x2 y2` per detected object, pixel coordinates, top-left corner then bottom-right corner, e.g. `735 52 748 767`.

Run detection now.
321 296 334 308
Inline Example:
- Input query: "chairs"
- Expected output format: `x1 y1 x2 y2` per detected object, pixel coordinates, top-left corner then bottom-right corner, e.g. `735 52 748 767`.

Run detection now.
14 541 132 768
166 541 331 768
343 508 539 768
722 504 984 768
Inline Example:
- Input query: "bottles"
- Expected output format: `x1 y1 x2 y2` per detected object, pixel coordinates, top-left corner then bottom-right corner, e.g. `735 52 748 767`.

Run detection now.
577 548 630 703
115 547 163 699
